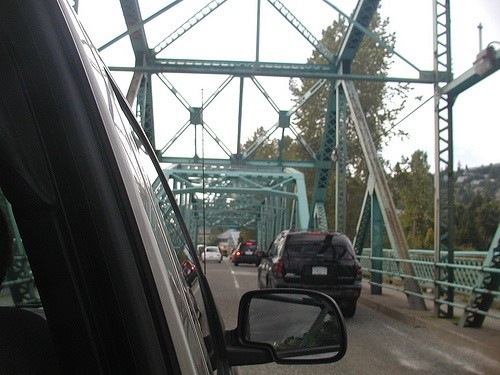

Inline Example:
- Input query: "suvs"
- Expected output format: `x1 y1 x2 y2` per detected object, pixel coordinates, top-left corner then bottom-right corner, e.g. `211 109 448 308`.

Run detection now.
181 259 198 288
254 229 364 319
229 242 263 267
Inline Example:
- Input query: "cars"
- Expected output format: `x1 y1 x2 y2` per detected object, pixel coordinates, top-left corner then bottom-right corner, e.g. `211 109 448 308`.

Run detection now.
197 245 222 263
221 248 229 258
